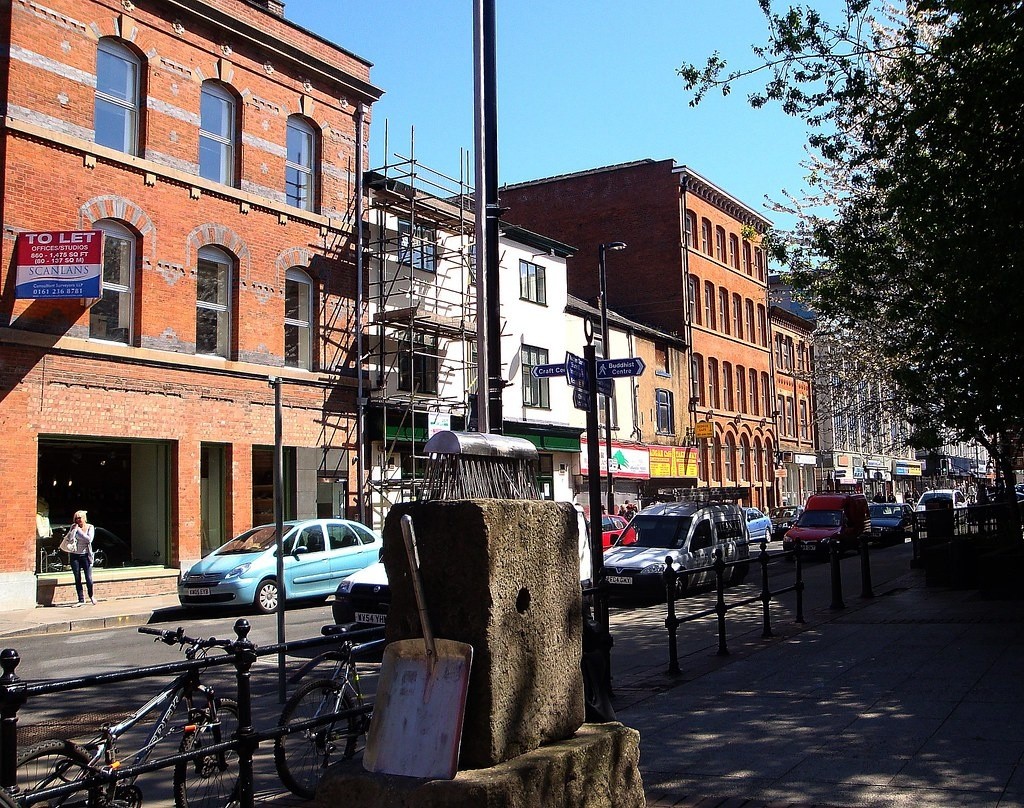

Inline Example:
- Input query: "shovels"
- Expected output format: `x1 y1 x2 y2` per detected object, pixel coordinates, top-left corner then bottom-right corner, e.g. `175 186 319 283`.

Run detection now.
353 506 483 783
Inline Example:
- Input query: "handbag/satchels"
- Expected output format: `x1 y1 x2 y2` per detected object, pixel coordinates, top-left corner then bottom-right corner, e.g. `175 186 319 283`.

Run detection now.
59 532 78 553
87 524 93 565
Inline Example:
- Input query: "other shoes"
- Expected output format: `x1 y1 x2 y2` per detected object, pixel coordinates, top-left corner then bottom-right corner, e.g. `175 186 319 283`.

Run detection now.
71 603 82 608
90 596 97 605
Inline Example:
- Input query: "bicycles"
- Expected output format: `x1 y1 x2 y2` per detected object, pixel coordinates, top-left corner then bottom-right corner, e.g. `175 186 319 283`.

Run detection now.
39 527 107 572
16 625 258 808
274 621 391 800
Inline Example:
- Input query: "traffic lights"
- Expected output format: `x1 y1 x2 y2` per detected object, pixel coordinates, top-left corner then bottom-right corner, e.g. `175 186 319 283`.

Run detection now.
940 459 948 476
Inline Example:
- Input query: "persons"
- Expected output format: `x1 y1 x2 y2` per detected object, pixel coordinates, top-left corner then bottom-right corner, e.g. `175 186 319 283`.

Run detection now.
36 490 53 572
912 483 976 503
976 485 989 533
888 492 896 503
633 504 638 513
625 506 634 522
873 492 883 503
904 489 911 503
618 505 626 516
70 510 97 608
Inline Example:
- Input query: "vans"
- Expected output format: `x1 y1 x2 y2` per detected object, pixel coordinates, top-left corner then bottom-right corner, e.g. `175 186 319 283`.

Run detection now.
602 499 750 601
781 490 871 556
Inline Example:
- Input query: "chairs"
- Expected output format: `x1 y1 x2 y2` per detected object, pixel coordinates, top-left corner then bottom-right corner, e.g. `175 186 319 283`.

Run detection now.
304 533 355 551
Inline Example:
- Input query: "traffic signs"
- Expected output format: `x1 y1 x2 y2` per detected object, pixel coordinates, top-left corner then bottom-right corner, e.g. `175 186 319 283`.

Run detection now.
531 350 646 412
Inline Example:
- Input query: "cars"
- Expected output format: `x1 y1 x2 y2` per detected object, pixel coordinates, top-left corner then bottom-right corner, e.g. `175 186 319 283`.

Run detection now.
867 501 918 548
178 519 385 614
35 525 131 570
585 515 637 552
741 507 773 544
332 546 391 625
767 505 803 540
915 489 969 530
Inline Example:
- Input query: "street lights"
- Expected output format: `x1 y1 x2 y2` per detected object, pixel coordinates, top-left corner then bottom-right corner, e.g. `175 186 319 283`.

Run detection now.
599 242 631 514
